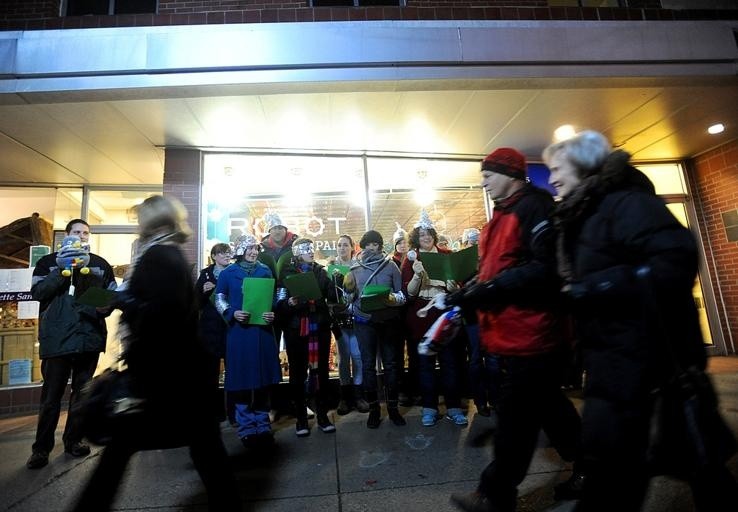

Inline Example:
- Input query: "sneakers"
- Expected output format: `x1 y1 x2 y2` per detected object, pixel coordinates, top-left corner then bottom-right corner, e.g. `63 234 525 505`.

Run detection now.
445 406 468 425
26 450 49 469
295 420 310 436
452 490 516 511
477 403 491 417
551 469 584 500
317 414 335 432
64 441 91 457
399 391 438 426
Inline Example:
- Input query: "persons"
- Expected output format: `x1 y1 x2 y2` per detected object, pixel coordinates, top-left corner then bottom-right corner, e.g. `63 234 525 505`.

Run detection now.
26 219 118 468
449 147 583 512
443 129 734 512
195 217 499 446
70 193 254 512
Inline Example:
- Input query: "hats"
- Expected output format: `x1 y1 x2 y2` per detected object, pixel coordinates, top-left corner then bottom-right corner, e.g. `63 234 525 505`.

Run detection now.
393 227 408 251
481 148 527 181
230 234 261 259
414 207 435 231
261 213 288 242
360 230 383 249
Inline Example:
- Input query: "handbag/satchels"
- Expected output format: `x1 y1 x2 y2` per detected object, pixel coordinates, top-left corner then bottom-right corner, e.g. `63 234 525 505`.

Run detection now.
641 370 738 480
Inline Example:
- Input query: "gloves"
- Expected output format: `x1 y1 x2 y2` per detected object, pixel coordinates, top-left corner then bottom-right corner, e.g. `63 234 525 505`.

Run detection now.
443 279 504 310
406 260 425 297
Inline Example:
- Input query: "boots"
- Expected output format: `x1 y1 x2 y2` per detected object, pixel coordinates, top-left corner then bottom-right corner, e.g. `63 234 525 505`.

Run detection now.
363 375 380 428
337 383 353 414
351 383 369 412
384 375 406 425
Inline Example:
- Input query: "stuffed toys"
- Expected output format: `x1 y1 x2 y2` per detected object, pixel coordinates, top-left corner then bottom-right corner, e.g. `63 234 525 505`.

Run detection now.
55 236 91 278
